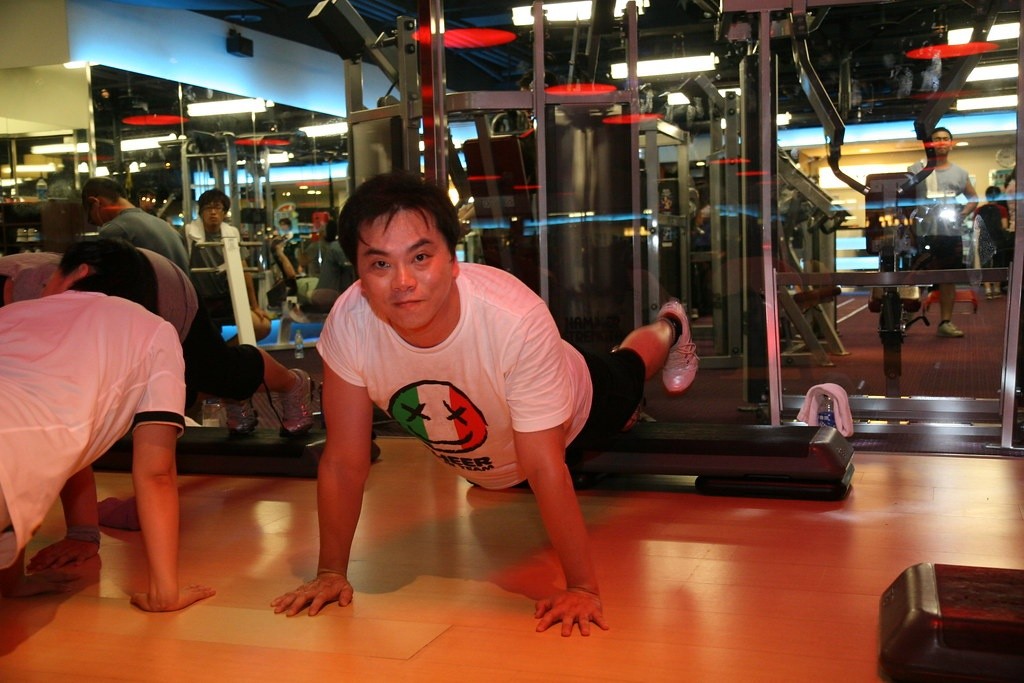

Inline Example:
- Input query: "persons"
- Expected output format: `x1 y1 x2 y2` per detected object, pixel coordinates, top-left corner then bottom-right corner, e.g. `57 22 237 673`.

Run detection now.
78 175 188 342
0 238 310 613
979 163 1017 294
276 213 299 299
192 189 268 329
270 170 699 637
909 128 980 341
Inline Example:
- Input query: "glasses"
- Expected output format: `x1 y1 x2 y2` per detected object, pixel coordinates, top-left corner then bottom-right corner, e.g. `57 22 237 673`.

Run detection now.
201 205 225 211
87 193 100 226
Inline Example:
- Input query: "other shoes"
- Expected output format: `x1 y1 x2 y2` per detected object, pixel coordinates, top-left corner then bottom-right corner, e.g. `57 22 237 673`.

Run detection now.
986 294 992 299
994 293 1000 298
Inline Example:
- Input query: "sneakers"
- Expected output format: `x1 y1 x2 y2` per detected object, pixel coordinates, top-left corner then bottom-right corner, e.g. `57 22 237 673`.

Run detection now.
936 321 964 337
225 395 258 437
279 369 314 433
655 301 701 396
